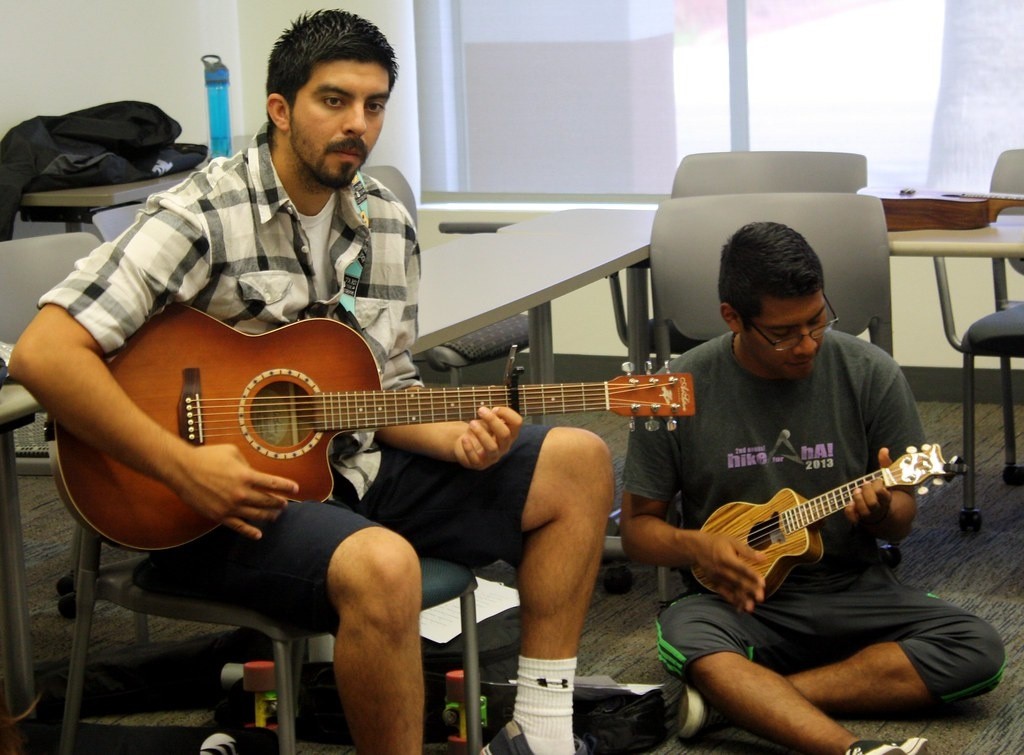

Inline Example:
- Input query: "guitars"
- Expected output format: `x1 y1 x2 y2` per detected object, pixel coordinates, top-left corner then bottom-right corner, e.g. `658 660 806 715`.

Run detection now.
47 299 697 553
858 188 1024 232
691 443 950 602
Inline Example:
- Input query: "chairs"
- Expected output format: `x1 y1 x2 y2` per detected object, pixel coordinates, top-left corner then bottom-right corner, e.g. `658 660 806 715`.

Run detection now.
0 147 1024 755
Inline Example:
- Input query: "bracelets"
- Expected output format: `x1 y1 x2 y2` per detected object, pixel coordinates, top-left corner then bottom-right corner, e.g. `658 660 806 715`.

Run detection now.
859 509 889 525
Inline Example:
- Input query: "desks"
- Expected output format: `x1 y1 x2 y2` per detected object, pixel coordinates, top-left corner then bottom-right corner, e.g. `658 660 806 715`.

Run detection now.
20 168 201 232
1 235 652 717
438 211 1024 408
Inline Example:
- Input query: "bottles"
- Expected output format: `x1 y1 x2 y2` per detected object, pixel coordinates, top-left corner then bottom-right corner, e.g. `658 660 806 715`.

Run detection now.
442 670 471 755
201 55 234 159
241 661 278 732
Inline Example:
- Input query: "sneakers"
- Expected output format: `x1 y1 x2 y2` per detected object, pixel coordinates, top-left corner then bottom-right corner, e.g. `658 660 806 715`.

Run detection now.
676 685 731 738
844 737 928 755
478 719 589 755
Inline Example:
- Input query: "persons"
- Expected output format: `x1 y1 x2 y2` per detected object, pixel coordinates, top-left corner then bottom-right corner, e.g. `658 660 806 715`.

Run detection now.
620 221 1006 755
8 8 615 755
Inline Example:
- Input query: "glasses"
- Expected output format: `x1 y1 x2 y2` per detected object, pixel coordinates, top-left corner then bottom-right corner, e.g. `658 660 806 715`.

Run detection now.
739 291 838 351
